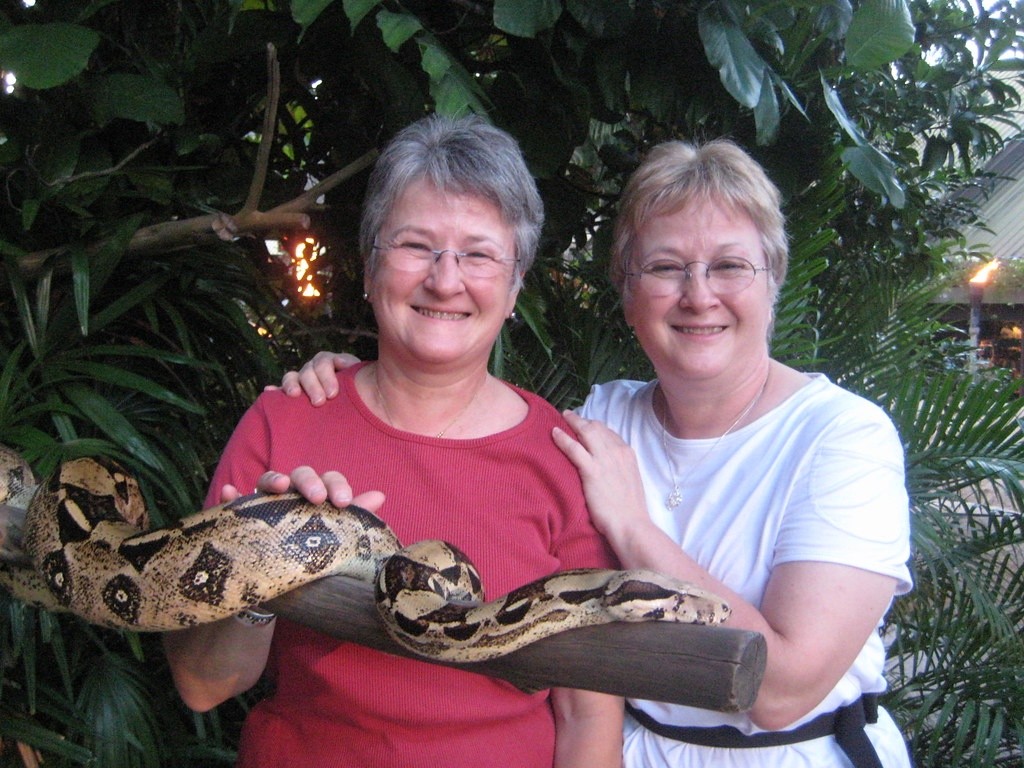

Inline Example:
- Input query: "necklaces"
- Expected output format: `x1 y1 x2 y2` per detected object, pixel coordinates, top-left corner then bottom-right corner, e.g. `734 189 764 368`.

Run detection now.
660 364 773 511
372 361 485 438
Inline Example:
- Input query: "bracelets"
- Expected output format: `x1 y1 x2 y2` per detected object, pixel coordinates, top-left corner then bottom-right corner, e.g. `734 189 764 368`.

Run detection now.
237 609 276 629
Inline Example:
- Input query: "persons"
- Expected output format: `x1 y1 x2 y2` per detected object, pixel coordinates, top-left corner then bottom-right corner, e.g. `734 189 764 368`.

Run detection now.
174 112 629 768
257 142 917 767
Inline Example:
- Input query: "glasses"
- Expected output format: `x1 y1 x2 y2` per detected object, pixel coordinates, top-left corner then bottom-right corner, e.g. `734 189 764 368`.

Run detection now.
372 234 520 278
625 256 771 296
